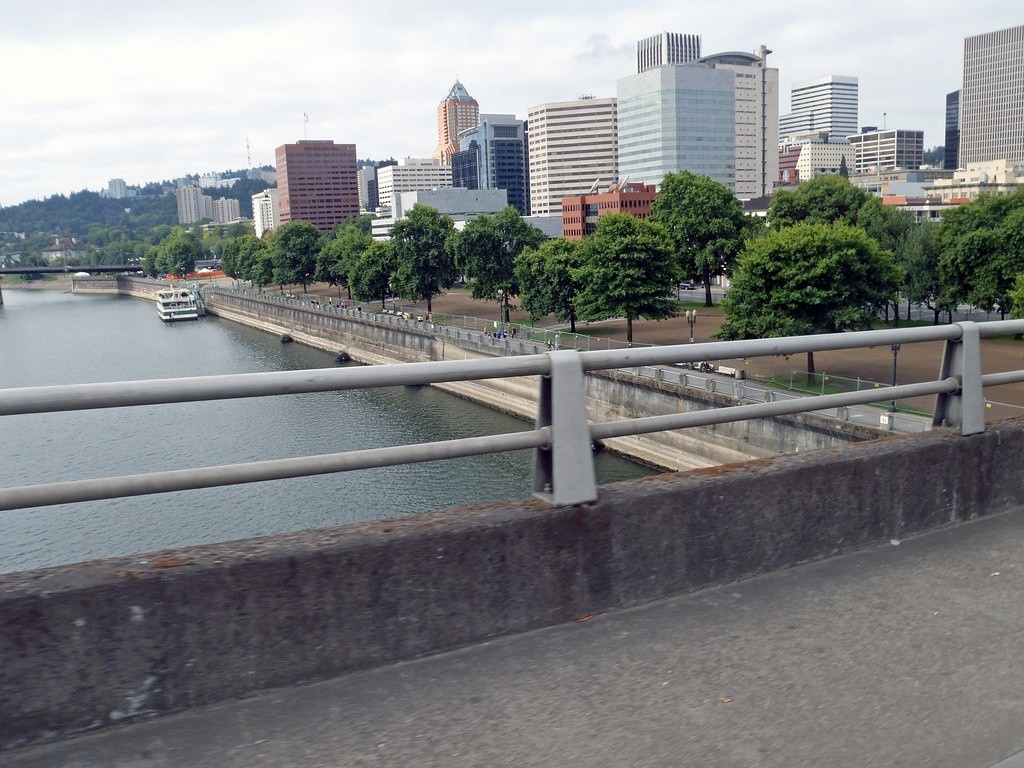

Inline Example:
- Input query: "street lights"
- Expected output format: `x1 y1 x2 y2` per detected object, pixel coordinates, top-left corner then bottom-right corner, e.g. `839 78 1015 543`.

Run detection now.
497 288 504 322
685 310 697 365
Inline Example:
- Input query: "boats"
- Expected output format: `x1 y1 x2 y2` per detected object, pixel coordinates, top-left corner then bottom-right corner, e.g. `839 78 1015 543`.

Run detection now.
156 284 199 321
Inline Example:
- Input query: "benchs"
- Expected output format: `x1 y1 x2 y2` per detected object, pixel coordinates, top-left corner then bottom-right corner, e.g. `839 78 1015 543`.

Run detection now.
381 309 412 318
672 361 736 376
